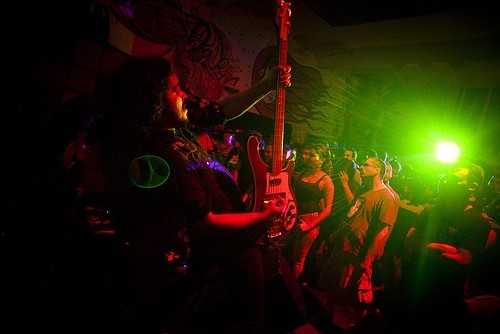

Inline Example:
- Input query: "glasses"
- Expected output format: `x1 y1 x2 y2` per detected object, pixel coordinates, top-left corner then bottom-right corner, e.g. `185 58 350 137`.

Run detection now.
360 163 377 169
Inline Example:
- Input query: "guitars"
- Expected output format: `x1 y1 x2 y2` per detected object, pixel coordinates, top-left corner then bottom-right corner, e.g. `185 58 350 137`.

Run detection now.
241 0 298 245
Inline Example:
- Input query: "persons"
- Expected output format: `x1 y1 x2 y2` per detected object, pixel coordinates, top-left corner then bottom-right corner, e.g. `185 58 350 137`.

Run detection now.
402 161 491 334
90 56 293 334
64 131 90 198
287 145 335 290
329 158 398 322
197 101 500 265
